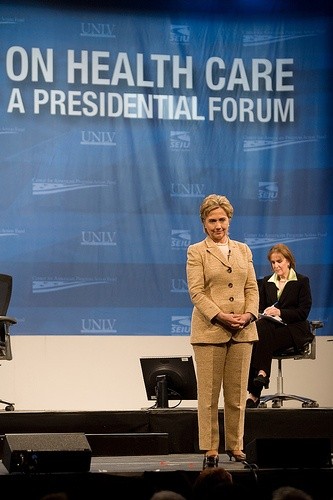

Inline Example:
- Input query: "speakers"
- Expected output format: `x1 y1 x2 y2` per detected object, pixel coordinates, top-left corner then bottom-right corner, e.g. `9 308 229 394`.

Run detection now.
243 436 333 468
0 432 92 472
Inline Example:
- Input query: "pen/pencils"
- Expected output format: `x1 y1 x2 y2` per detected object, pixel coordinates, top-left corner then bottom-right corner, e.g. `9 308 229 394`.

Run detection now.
264 302 278 315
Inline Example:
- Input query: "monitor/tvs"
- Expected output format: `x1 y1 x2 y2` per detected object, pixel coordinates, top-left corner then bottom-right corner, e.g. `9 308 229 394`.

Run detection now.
139 355 198 410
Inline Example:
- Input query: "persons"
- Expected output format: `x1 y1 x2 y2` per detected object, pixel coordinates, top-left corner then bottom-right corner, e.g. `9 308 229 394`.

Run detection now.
152 467 310 500
245 243 313 408
186 194 259 469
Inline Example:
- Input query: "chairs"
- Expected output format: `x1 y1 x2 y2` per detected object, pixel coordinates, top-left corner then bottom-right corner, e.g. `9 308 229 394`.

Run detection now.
257 279 324 407
0 273 18 411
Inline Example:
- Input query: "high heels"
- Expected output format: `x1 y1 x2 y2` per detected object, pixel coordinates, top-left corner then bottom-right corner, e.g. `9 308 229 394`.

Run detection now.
246 397 260 408
254 374 270 389
227 450 246 461
203 453 219 471
